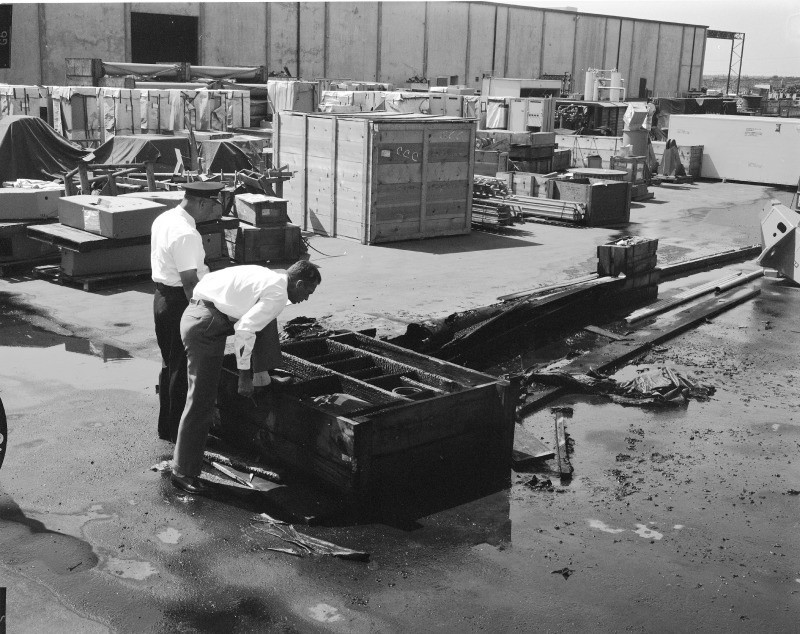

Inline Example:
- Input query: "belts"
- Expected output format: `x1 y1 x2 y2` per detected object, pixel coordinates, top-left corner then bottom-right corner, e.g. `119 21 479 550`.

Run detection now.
189 299 215 308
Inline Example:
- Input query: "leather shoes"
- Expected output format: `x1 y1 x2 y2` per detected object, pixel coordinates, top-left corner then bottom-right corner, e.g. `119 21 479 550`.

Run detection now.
170 473 216 495
254 376 292 393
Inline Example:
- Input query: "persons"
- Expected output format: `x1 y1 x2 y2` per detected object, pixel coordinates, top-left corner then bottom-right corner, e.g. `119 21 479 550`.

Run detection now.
151 181 251 440
171 259 321 499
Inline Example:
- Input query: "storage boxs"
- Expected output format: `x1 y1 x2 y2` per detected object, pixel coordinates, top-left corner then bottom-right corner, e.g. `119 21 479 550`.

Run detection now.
201 89 229 133
0 184 64 223
136 88 171 135
221 217 304 264
663 111 800 190
473 96 649 227
164 87 201 133
265 110 481 249
334 80 393 92
314 78 353 92
263 76 320 117
382 90 430 115
65 74 99 87
213 326 522 524
116 189 186 211
678 142 707 182
55 193 168 242
97 86 143 137
594 234 660 273
45 84 99 141
62 56 104 78
220 88 252 128
319 88 388 112
0 83 42 121
102 75 136 90
424 91 464 117
234 192 290 227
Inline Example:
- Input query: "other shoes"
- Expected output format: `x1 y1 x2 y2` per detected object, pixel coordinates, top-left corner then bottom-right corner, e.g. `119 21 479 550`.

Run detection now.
205 436 216 446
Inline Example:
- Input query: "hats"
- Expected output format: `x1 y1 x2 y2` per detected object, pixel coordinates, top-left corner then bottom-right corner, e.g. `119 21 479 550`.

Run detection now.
181 181 225 204
